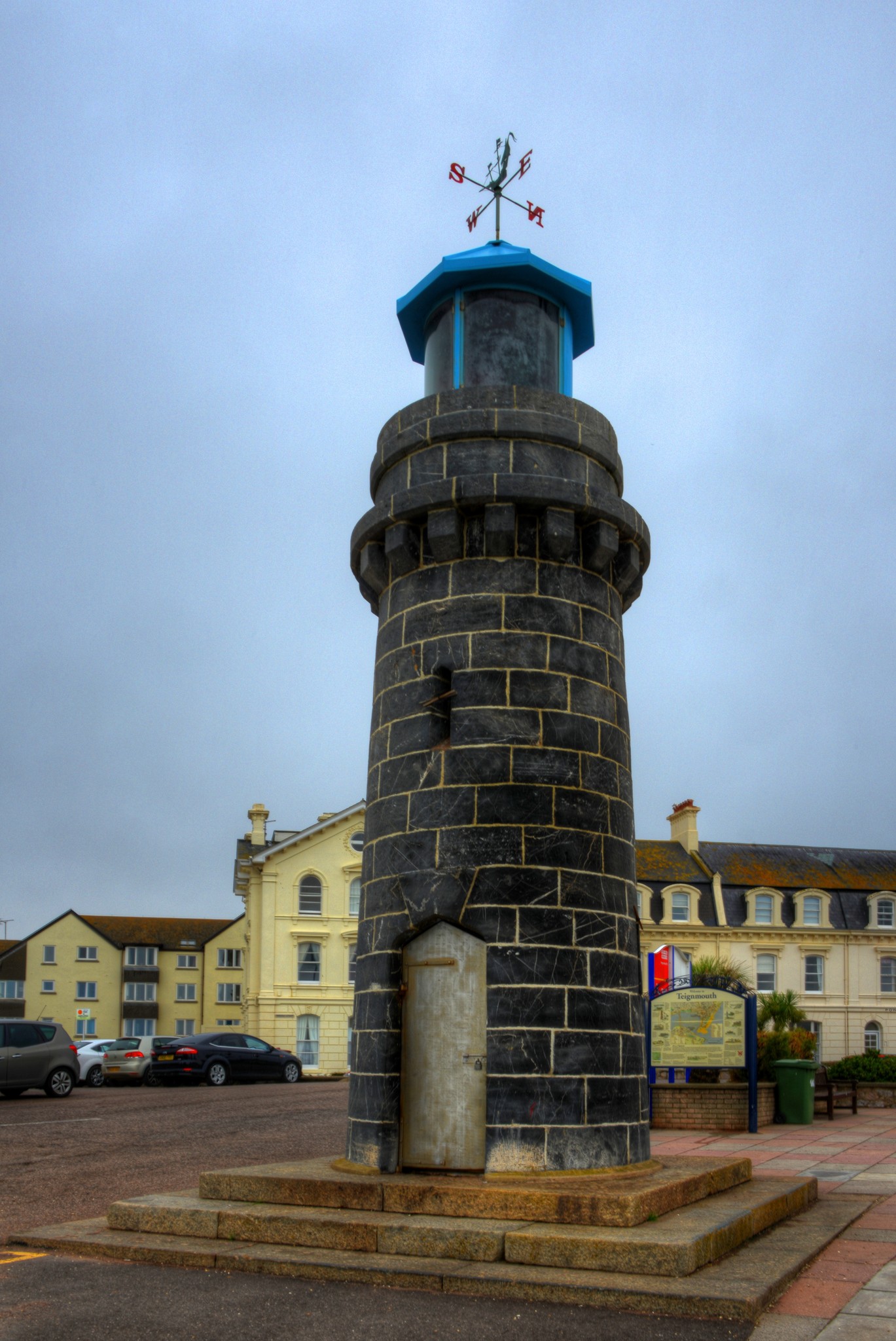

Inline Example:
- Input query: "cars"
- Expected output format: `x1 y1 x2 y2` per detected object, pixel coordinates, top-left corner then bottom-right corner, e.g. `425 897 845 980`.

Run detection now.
73 1038 139 1088
101 1034 183 1086
149 1032 303 1086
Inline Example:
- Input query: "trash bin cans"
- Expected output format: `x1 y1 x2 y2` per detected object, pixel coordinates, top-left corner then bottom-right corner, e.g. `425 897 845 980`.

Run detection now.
775 1058 821 1124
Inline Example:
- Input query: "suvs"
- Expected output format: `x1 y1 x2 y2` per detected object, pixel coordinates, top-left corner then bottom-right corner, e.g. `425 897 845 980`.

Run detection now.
0 1019 81 1099
